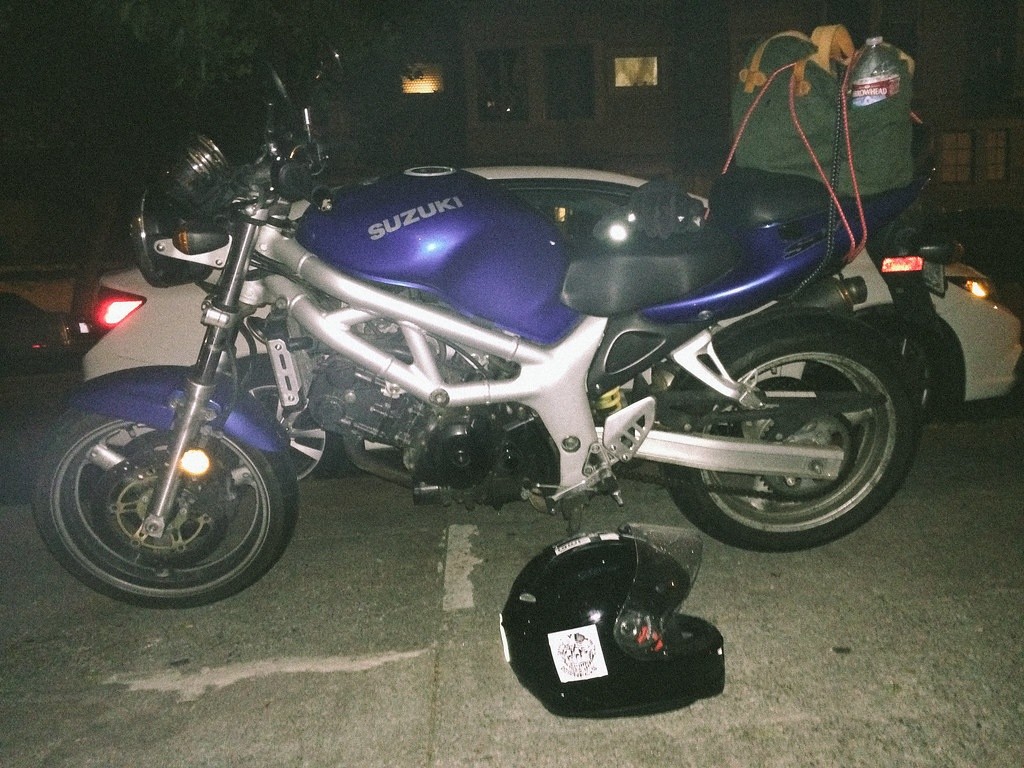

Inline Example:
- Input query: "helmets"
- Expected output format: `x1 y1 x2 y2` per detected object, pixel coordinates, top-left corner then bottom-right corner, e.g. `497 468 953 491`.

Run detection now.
504 523 727 719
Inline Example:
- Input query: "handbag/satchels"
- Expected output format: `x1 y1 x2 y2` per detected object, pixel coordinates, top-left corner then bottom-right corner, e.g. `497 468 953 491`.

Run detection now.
734 19 915 197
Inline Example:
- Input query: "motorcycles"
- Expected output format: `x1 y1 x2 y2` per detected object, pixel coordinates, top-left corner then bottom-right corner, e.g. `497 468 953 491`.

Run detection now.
24 37 943 610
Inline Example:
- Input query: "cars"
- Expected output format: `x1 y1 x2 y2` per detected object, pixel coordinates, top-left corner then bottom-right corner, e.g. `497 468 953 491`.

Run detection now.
65 165 1024 481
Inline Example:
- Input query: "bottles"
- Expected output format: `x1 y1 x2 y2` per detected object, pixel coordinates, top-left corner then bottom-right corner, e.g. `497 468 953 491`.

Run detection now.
847 36 901 106
